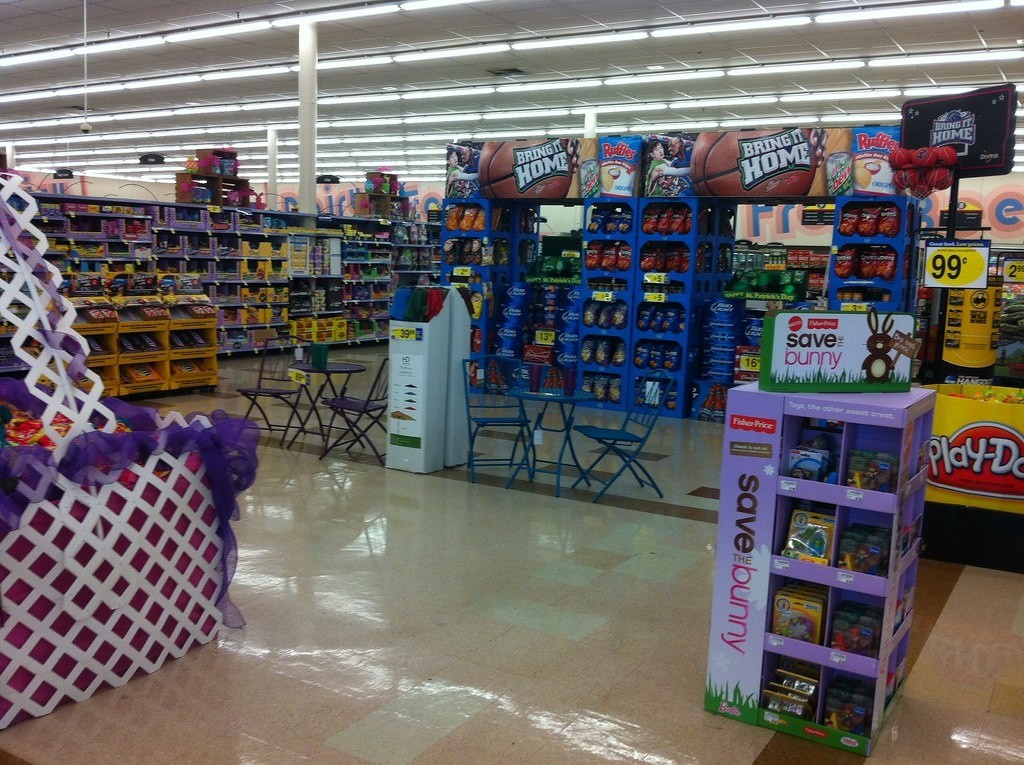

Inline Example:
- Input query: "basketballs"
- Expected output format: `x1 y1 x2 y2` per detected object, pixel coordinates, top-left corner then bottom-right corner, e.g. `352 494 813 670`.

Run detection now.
690 129 819 196
888 147 958 199
478 138 574 198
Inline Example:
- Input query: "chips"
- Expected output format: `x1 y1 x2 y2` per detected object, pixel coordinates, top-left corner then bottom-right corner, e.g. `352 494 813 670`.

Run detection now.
853 161 871 187
602 167 613 192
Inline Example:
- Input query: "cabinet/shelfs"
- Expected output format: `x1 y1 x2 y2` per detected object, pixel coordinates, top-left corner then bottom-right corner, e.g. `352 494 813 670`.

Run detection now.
701 380 937 757
0 192 1024 421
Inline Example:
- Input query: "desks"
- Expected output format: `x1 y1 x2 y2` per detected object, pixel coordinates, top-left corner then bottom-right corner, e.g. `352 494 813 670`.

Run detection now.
504 387 595 490
288 362 366 452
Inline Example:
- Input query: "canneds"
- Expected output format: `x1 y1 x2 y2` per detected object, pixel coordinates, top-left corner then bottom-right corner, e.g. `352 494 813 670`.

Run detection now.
826 152 853 196
580 159 600 198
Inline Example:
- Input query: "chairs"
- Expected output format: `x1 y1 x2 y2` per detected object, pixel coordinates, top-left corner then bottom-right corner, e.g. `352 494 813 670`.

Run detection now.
319 358 389 466
462 355 535 484
572 369 673 504
237 335 307 446
